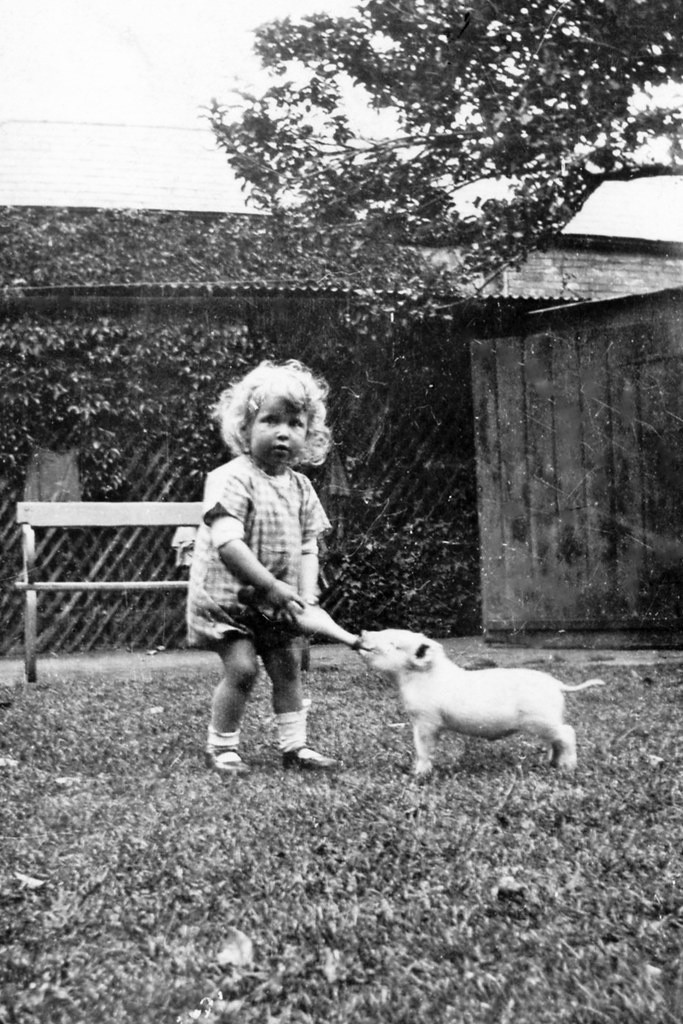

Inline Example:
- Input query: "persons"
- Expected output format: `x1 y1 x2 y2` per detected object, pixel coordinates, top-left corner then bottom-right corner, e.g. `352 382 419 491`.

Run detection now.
183 356 340 777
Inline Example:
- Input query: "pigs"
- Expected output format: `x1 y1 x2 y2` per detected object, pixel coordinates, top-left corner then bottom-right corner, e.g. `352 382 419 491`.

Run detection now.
359 629 606 775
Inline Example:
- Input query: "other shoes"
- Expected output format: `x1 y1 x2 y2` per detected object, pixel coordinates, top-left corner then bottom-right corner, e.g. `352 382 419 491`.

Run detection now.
280 746 347 773
203 747 252 777
